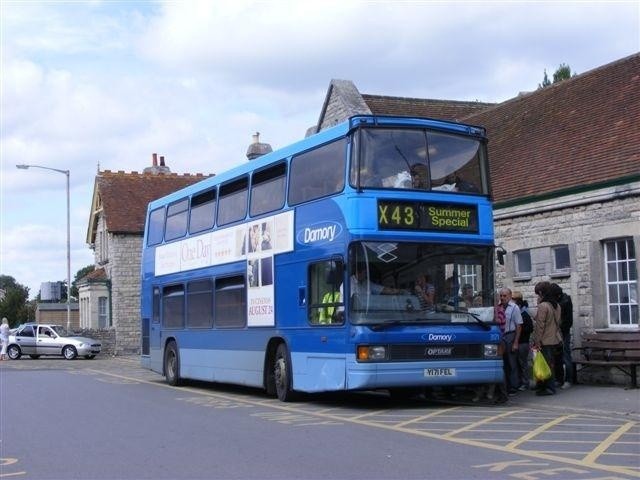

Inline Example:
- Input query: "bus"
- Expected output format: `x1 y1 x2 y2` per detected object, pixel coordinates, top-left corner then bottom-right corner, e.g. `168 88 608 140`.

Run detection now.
139 111 509 402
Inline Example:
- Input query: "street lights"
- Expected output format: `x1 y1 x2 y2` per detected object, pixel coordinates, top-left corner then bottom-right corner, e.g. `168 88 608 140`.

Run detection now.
16 164 72 336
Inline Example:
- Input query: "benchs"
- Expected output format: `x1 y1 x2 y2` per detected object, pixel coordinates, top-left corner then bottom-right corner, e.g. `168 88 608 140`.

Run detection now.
568 329 639 388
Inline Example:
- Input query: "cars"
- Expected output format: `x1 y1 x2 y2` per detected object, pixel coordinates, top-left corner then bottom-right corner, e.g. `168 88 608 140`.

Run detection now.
7 322 102 360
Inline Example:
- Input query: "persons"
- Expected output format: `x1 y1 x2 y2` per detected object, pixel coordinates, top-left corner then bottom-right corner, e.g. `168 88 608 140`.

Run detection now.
358 157 479 195
0 317 13 362
248 220 271 288
316 261 481 326
480 282 577 407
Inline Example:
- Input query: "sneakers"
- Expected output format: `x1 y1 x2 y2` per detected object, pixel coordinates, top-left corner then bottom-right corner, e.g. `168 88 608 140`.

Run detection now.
508 391 518 397
532 380 573 396
518 386 527 391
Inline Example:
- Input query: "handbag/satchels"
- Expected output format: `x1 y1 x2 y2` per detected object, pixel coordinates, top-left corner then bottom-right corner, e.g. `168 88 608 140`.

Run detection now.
556 328 564 341
